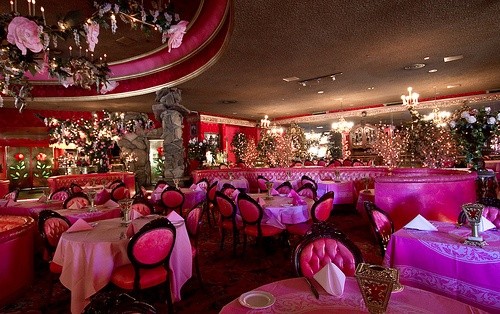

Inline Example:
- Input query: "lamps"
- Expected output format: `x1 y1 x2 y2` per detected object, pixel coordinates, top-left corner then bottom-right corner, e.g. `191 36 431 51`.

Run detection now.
0 1 499 162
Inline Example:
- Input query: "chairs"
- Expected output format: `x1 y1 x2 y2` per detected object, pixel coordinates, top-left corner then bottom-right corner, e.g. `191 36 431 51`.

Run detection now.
1 172 500 312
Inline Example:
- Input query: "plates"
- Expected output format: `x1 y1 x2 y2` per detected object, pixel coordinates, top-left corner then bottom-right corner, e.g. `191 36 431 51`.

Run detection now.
238 291 276 309
280 204 293 207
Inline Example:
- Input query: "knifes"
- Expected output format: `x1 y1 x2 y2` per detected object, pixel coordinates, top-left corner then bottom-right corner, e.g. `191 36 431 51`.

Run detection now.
304 277 319 300
